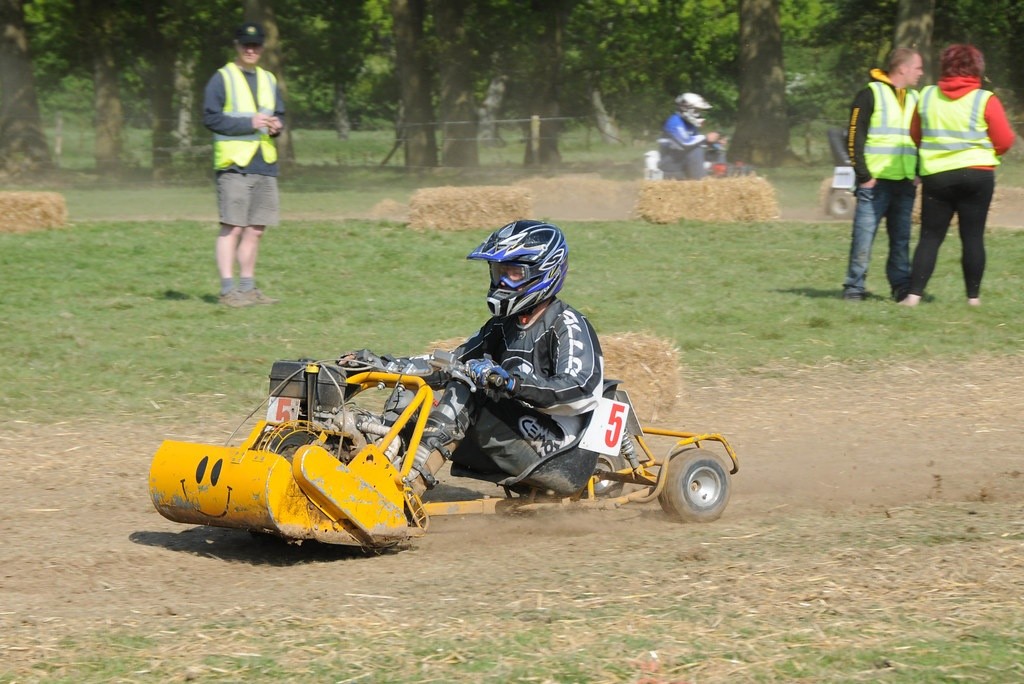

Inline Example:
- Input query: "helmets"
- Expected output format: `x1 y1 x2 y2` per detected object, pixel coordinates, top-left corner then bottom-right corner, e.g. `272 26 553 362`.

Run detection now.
672 93 713 127
466 220 568 319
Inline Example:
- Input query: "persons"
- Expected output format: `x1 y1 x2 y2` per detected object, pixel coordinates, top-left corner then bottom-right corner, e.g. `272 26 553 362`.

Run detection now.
842 51 937 304
336 219 603 508
661 90 729 180
201 23 287 305
896 43 1016 305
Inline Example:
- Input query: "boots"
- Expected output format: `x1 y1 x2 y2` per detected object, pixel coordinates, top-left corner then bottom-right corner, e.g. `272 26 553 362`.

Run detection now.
400 438 460 498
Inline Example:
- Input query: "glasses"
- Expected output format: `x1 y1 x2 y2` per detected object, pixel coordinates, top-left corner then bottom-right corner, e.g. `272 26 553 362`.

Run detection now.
488 259 530 289
689 108 701 117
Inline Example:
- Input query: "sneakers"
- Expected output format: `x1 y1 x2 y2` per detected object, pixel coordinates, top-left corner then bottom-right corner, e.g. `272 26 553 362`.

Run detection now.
218 290 255 308
239 286 280 304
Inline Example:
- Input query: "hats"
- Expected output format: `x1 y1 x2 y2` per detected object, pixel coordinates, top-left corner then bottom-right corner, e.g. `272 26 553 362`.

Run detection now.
235 23 264 44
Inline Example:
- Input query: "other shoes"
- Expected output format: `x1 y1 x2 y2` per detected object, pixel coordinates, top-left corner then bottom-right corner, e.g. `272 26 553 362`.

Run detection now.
842 286 864 300
892 283 912 301
968 297 981 306
897 294 921 307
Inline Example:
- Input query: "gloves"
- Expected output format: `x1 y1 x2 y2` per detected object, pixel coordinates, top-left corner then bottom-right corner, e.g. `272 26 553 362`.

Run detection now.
465 358 520 396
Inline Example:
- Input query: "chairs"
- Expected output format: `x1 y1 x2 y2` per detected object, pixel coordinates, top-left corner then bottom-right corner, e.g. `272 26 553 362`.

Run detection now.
656 138 720 181
828 130 852 166
450 378 624 496
